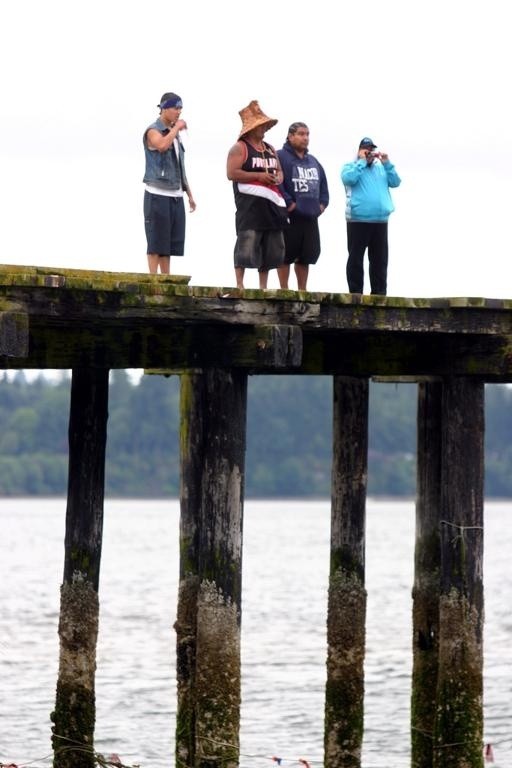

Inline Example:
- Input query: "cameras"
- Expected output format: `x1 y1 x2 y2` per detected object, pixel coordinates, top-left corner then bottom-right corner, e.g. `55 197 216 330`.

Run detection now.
365 150 380 158
271 176 278 184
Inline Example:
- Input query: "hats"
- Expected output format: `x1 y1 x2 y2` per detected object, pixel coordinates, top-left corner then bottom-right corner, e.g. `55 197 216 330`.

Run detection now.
238 99 278 140
360 137 378 149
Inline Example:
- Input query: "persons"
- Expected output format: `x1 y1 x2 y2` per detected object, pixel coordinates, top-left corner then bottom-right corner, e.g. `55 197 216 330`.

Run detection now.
226 99 284 290
341 135 402 297
140 91 197 275
277 121 329 290
